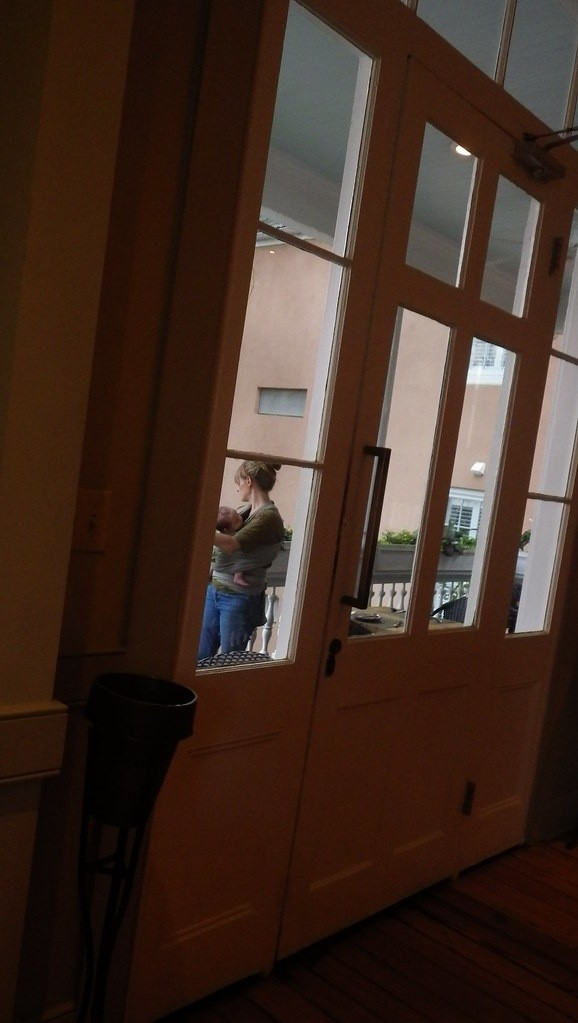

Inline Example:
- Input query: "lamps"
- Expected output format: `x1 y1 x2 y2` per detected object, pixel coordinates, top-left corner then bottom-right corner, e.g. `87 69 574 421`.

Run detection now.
470 462 487 477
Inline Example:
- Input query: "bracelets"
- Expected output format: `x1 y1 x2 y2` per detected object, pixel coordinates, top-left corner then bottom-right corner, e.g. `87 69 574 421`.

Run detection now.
215 531 220 546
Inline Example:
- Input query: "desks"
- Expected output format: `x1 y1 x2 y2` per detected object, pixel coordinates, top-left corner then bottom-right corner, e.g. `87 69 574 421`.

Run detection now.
350 606 464 633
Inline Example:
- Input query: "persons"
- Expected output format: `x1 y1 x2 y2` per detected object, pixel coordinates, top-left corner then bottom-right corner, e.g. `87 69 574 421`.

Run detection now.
199 461 284 661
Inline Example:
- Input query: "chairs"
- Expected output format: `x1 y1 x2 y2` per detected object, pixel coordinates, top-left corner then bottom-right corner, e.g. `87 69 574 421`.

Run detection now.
432 597 467 623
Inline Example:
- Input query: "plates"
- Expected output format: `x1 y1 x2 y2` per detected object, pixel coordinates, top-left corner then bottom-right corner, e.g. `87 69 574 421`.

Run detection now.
357 613 381 620
